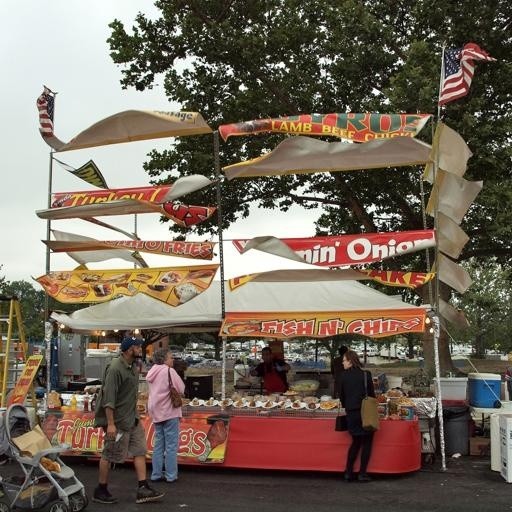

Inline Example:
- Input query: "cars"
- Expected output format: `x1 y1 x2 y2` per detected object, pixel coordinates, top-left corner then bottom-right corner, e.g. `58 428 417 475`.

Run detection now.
283 340 423 366
174 341 266 362
450 342 473 356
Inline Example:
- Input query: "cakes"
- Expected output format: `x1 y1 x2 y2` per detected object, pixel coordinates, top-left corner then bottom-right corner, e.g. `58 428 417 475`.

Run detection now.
192 396 199 405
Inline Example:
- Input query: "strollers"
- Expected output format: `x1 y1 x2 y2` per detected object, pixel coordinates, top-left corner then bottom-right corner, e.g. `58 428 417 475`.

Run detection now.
0 405 88 512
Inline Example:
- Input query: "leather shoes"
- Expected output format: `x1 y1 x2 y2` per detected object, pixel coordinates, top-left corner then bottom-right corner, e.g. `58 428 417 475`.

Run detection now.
335 471 373 483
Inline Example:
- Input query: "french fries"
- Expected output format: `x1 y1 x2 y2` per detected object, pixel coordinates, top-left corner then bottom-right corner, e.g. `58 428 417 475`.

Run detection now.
320 401 335 409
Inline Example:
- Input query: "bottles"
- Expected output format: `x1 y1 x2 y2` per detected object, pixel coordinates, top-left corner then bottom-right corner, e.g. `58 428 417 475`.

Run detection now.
69 393 98 413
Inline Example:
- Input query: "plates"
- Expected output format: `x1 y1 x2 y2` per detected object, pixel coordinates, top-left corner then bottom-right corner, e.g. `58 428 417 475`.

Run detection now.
189 390 339 411
378 395 414 408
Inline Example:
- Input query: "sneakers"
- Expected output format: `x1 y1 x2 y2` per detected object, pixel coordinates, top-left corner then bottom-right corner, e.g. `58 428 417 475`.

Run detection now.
135 485 165 504
91 486 119 504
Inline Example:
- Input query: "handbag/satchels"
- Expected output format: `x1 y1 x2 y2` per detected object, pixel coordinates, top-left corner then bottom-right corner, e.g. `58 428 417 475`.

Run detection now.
335 414 348 432
167 366 183 408
361 369 381 433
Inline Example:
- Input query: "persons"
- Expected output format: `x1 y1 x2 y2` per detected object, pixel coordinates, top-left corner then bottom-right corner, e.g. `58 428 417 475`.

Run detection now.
92 338 166 504
331 346 347 398
145 347 186 483
172 353 187 381
336 351 377 482
249 348 291 396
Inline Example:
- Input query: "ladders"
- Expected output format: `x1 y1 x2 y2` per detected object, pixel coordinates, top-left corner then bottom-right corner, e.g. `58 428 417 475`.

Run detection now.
0 295 41 428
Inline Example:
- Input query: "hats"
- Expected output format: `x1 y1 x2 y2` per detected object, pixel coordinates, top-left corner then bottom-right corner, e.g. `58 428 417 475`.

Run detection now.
120 335 144 353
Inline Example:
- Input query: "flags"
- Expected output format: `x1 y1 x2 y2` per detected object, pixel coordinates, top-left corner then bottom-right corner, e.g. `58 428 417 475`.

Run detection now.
36 85 54 133
439 42 498 105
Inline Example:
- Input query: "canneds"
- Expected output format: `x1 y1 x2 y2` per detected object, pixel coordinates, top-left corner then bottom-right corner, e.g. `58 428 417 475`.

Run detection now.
210 397 214 405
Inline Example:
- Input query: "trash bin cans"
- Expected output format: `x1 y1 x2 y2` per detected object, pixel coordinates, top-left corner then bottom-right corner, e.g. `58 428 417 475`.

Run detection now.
435 406 469 457
